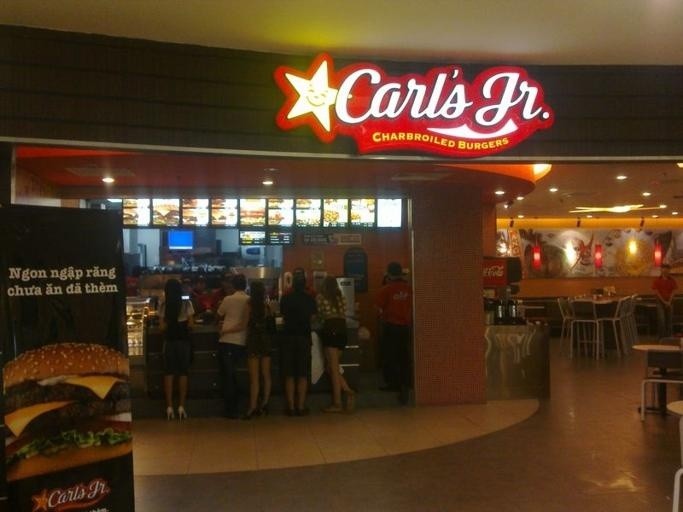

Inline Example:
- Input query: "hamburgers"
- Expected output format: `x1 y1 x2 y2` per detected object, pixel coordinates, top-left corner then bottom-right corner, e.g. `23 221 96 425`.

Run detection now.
1 342 133 482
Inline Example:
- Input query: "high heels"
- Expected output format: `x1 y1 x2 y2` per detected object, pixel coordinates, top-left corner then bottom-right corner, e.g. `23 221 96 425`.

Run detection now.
178 406 187 420
166 407 175 420
244 402 267 420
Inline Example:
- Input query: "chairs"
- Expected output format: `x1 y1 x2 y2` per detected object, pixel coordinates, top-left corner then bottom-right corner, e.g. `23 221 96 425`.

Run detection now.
631 337 682 422
556 294 639 362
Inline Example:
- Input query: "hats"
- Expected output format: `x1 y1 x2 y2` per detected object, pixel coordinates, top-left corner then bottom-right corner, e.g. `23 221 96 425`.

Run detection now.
388 262 406 276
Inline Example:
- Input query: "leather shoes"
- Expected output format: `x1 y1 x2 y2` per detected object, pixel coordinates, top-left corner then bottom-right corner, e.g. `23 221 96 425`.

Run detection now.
288 408 307 416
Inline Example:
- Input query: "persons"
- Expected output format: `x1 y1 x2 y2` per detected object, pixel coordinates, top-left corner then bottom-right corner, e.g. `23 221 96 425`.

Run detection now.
373 261 412 393
187 273 213 314
213 274 250 419
126 262 147 296
275 267 317 416
155 278 198 422
314 275 356 414
218 281 277 421
650 262 679 343
281 267 317 301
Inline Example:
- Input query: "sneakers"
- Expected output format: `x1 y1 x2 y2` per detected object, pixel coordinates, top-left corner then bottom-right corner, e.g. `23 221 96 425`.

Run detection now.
322 405 344 413
347 391 355 411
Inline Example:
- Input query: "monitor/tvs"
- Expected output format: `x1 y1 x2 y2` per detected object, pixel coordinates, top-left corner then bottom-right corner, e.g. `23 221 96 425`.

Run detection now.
166 228 196 252
